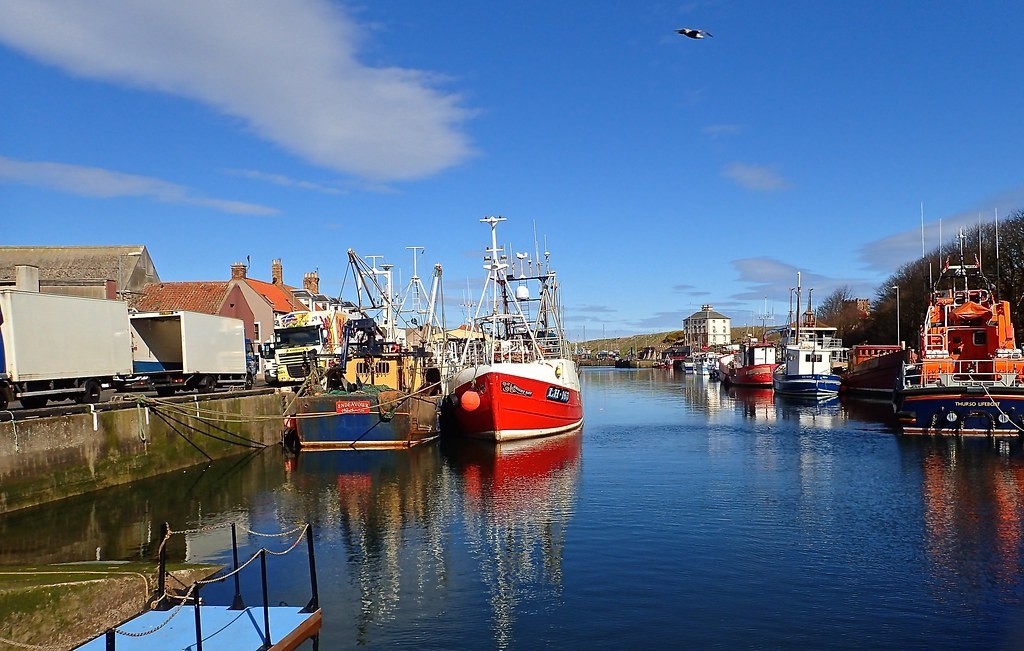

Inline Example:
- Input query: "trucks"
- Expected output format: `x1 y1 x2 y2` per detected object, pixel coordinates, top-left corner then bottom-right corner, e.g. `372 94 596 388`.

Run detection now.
0 291 135 411
129 310 258 394
257 310 357 398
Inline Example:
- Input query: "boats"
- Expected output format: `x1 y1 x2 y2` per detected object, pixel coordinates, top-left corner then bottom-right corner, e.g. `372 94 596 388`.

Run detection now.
841 341 920 401
773 271 844 395
614 296 778 385
891 202 1024 441
281 248 450 452
451 215 582 442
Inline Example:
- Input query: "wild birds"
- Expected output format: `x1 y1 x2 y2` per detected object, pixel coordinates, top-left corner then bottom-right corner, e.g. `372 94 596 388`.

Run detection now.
673 27 714 40
